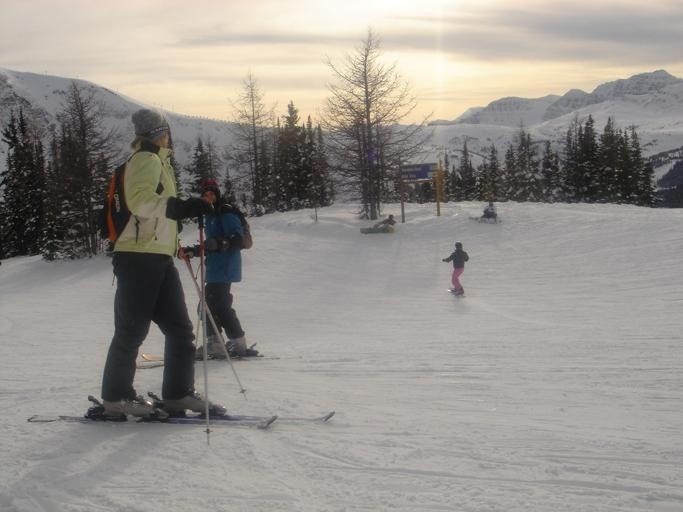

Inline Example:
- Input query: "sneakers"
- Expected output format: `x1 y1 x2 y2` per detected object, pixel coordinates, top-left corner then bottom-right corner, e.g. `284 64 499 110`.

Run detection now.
103 395 169 419
162 388 227 416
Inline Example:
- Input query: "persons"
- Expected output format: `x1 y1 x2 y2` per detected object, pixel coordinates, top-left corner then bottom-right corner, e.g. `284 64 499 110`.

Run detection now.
95 108 226 421
373 215 395 231
180 180 252 360
441 241 468 296
479 200 497 219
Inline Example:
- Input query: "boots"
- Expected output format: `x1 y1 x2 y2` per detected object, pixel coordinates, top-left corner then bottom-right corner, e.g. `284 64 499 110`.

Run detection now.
224 336 247 357
194 334 226 360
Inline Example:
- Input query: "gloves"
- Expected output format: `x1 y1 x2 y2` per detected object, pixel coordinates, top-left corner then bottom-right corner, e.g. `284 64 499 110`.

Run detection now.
205 238 229 252
166 196 212 220
177 245 198 259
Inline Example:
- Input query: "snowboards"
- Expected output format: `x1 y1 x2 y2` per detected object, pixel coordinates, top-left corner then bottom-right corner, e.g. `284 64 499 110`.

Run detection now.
359 227 392 232
448 287 466 299
468 216 496 224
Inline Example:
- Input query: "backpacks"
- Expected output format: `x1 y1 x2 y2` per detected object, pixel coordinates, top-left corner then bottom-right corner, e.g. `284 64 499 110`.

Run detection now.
94 147 165 252
213 203 252 250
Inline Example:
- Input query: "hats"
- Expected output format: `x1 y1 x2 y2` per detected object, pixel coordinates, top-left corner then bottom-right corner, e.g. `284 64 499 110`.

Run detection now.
199 180 220 199
132 109 169 138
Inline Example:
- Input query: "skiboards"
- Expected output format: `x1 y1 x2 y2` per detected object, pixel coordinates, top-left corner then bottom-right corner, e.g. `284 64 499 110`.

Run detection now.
27 391 336 430
135 343 302 367
